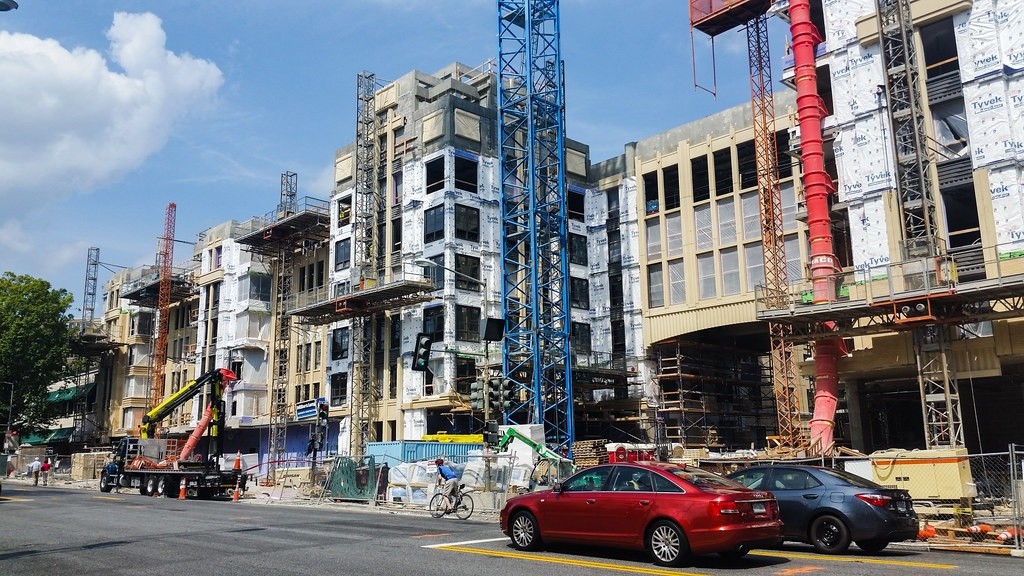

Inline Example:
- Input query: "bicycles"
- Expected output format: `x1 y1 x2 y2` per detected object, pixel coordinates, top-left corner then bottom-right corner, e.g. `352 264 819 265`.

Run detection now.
428 477 475 520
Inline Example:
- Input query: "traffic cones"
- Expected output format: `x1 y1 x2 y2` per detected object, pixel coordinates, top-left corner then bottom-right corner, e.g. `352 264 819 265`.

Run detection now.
230 481 242 503
968 524 1023 542
177 478 187 500
232 449 242 470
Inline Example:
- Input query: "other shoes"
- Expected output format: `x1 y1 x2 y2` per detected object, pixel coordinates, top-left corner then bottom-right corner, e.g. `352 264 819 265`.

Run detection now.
446 510 452 514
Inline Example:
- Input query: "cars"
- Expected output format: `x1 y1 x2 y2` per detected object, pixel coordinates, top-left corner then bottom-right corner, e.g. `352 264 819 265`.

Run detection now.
723 465 919 554
499 460 787 565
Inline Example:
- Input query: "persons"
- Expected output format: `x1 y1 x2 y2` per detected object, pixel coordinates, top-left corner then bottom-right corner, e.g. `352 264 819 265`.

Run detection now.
435 459 458 515
31 457 50 486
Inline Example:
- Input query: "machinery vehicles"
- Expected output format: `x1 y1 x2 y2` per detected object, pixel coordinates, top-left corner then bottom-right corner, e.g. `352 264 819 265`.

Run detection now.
490 427 580 475
100 368 248 500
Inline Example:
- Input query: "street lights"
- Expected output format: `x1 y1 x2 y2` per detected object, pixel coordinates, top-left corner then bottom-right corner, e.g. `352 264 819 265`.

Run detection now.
0 381 15 454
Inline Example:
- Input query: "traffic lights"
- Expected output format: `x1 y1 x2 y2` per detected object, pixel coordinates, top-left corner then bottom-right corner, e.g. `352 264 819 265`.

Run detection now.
411 332 433 371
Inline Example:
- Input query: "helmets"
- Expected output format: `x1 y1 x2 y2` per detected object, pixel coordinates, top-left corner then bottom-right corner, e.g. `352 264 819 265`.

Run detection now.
435 459 443 464
